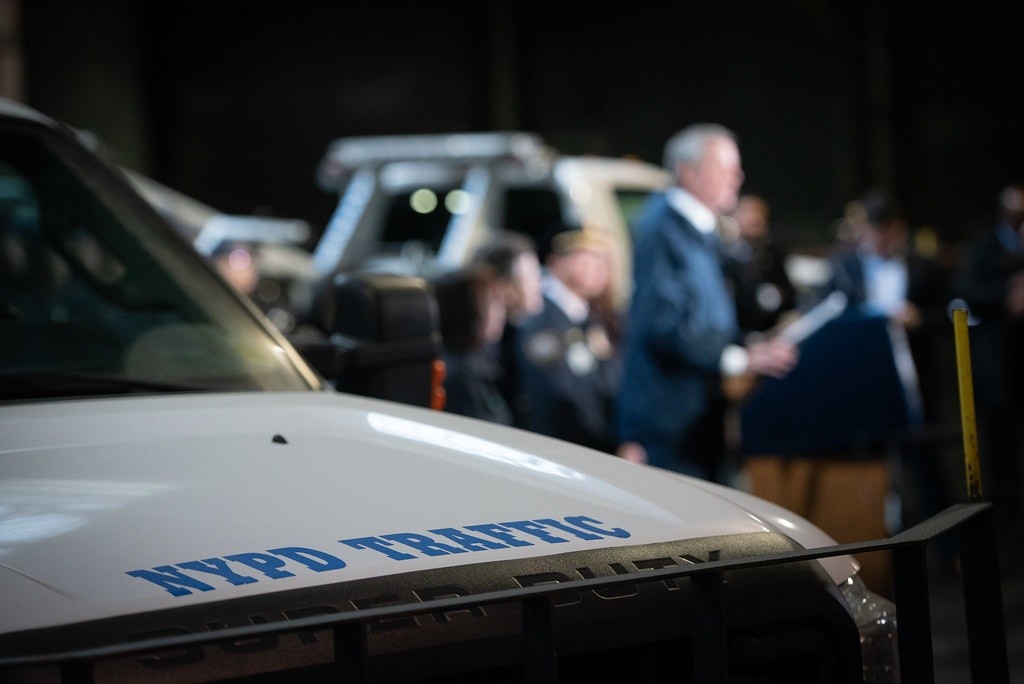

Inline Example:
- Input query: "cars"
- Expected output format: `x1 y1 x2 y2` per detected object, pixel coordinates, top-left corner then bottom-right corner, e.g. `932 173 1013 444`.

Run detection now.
0 101 902 684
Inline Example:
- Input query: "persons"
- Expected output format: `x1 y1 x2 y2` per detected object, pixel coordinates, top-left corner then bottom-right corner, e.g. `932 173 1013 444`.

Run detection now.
611 120 803 485
212 187 1023 586
